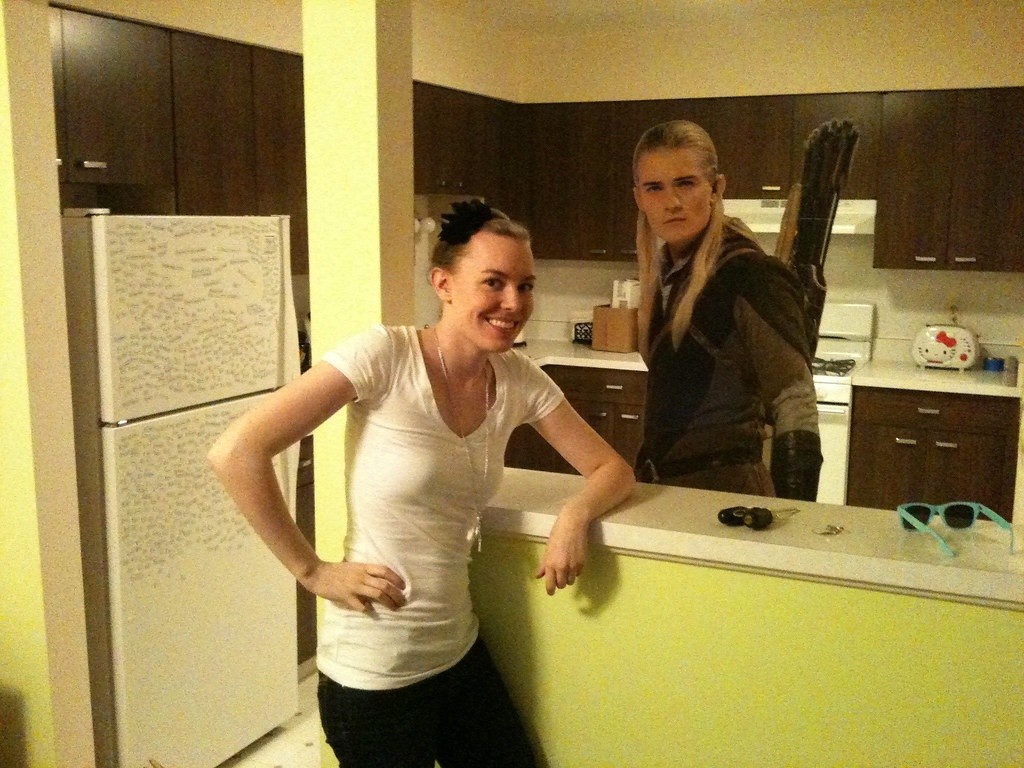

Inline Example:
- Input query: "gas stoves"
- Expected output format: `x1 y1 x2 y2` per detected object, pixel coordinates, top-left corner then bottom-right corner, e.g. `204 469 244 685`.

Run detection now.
812 304 875 404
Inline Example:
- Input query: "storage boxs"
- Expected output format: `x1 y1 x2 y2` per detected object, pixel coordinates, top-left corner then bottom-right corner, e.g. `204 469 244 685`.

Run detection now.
593 305 640 353
612 280 640 307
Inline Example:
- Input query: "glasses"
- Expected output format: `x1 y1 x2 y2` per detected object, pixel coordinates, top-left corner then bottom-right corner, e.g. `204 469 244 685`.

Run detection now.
897 501 1013 558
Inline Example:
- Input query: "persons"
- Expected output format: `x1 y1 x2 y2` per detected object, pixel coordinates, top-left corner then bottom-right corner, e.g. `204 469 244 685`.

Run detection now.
632 119 824 503
209 196 636 768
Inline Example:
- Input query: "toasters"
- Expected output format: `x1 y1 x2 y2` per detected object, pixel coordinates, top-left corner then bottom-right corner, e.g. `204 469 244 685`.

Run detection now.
912 324 980 371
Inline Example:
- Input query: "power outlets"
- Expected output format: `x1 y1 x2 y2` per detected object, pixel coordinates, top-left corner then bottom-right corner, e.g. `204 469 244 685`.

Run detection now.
947 302 960 324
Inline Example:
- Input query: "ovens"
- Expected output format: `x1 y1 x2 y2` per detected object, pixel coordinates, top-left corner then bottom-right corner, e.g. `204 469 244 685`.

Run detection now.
763 404 854 505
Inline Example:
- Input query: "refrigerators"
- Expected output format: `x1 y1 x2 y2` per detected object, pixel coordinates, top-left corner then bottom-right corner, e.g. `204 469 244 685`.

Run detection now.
61 209 302 768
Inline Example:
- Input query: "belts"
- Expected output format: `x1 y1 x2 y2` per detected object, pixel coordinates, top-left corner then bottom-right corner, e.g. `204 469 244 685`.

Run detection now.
635 445 764 482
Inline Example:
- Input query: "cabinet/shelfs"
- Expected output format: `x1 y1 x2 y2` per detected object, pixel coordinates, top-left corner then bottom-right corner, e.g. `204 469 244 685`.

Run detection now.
847 385 1020 523
502 364 649 477
50 0 309 274
413 79 1024 271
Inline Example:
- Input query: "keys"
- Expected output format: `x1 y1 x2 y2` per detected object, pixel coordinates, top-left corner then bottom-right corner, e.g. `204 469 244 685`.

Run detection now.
718 506 800 530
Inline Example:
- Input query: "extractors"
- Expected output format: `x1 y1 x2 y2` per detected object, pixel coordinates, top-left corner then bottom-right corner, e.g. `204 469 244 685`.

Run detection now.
721 199 878 235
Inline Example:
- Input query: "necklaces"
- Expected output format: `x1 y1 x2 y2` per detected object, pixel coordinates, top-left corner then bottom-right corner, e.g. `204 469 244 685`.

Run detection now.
434 328 489 552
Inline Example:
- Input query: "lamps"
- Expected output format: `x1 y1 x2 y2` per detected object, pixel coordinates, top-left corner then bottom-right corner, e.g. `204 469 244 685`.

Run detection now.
414 217 437 234
